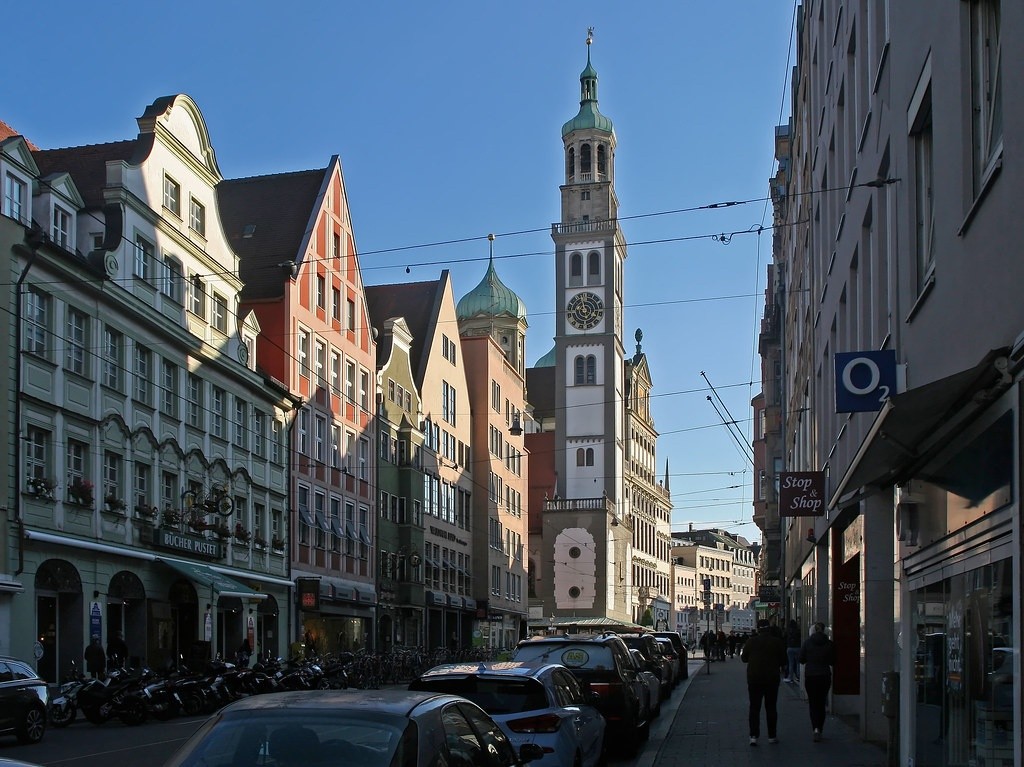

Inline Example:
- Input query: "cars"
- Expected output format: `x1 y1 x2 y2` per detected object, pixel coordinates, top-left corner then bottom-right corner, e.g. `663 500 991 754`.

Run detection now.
627 649 664 718
159 687 526 767
655 637 683 689
407 661 609 767
917 632 1013 731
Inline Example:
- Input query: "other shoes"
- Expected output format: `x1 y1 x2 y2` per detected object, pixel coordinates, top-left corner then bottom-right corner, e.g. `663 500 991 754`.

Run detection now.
783 678 791 682
933 736 945 746
768 738 777 744
749 739 757 745
813 728 821 742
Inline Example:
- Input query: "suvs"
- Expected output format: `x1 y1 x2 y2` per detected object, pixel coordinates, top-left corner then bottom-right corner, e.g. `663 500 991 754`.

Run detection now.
645 631 690 678
509 630 656 761
612 630 675 700
0 655 50 745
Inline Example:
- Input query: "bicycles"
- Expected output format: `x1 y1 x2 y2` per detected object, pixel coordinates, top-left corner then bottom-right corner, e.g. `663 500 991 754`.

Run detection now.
352 646 496 690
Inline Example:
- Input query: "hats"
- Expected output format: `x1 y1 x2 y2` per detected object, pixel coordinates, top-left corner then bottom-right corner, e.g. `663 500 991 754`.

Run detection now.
90 634 102 639
113 631 123 636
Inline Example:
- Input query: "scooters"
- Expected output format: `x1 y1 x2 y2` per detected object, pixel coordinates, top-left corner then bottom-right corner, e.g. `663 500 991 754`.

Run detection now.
50 648 351 726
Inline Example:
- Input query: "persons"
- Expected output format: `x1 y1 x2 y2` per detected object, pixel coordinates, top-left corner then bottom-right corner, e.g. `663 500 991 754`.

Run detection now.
741 615 790 746
106 631 128 667
84 634 106 682
700 630 756 661
237 639 253 668
785 619 800 683
799 623 837 743
